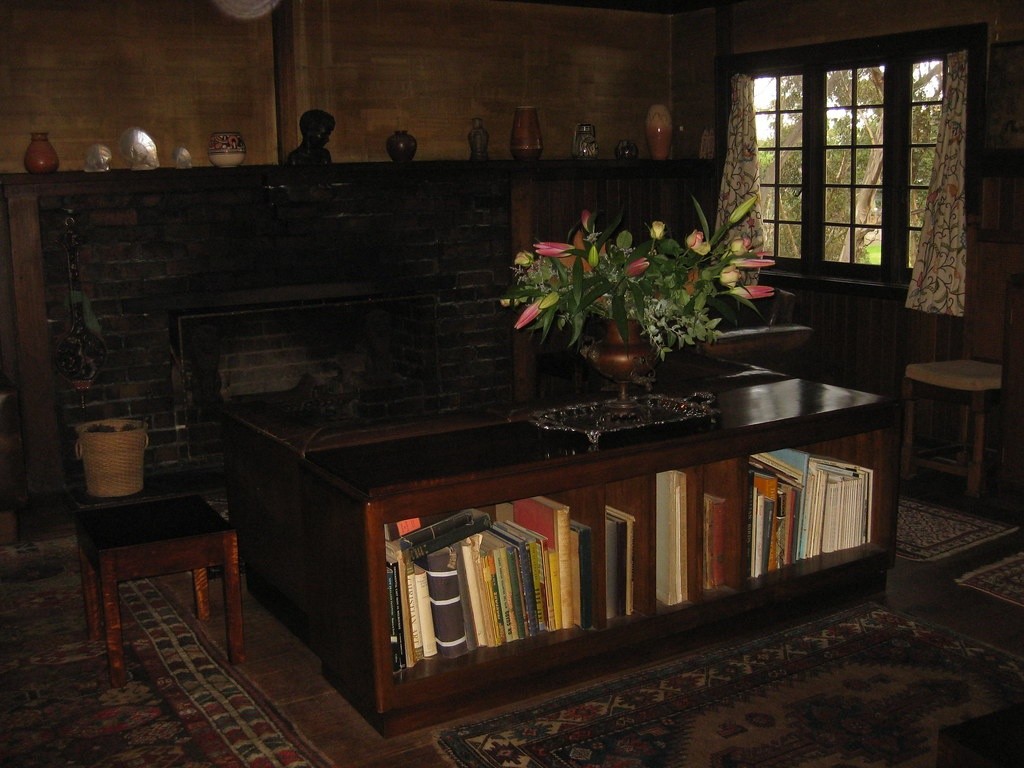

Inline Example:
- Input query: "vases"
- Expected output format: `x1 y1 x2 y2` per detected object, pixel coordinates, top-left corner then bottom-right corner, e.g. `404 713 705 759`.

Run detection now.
578 315 655 417
615 139 637 161
510 106 542 161
573 122 599 160
469 118 489 162
645 103 673 160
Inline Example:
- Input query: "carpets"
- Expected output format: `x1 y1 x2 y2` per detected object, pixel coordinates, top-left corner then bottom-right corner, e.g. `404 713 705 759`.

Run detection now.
0 532 338 768
434 598 1024 768
896 494 1020 562
953 552 1024 610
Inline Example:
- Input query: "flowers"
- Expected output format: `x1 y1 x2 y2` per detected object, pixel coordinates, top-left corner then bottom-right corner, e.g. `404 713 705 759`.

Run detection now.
497 195 777 362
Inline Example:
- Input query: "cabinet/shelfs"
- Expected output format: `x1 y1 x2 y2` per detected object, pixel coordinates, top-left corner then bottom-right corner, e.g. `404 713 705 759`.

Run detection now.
299 375 896 741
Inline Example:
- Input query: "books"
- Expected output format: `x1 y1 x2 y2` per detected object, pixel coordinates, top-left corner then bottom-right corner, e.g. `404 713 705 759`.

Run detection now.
745 447 870 580
513 495 574 629
384 508 562 674
605 504 636 620
569 519 593 631
656 470 726 605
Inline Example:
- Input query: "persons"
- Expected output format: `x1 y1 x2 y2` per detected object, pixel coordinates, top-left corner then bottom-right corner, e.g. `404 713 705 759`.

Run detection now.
289 109 336 164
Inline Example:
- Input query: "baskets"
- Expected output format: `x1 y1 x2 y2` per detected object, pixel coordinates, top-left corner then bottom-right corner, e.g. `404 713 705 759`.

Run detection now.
74 418 149 497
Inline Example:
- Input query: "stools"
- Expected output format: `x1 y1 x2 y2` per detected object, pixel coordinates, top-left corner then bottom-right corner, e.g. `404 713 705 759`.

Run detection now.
900 360 1002 509
73 494 243 688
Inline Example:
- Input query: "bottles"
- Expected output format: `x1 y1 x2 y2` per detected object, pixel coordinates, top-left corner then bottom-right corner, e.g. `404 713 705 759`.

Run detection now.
509 106 544 161
385 130 417 162
24 132 58 174
209 132 246 167
645 105 672 160
572 122 599 158
